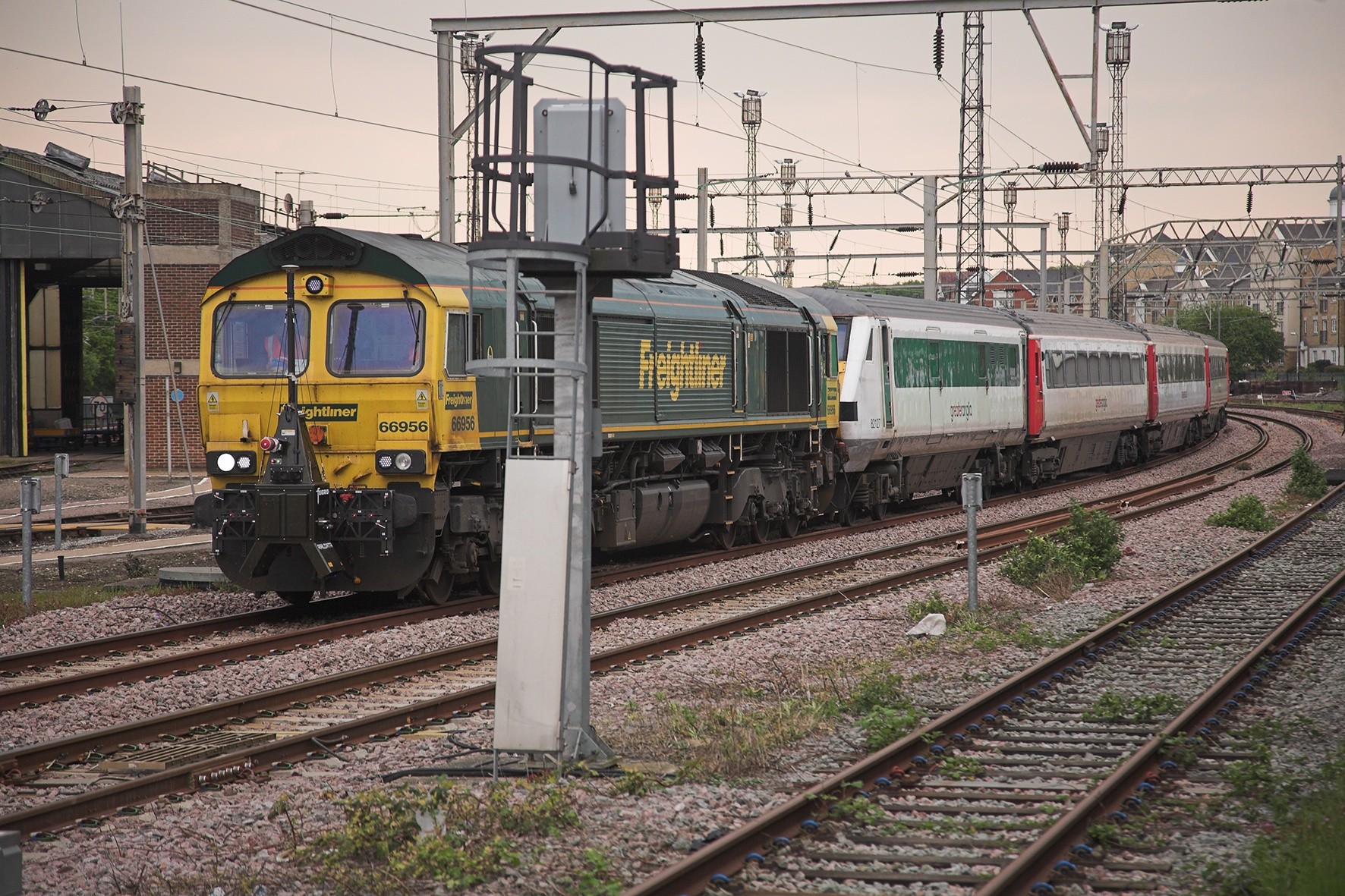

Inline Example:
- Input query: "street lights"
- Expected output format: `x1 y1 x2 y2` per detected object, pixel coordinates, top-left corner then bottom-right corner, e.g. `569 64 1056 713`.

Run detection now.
1336 282 1340 366
1291 332 1299 381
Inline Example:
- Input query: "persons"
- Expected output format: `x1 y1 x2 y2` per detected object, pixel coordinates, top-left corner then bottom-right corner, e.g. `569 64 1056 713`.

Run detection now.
256 315 308 371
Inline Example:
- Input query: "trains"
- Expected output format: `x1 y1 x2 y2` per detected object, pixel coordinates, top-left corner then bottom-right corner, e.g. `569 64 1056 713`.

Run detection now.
194 226 1229 603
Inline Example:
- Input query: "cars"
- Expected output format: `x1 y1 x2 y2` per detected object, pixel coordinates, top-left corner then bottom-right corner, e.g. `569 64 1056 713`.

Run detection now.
1243 359 1273 371
